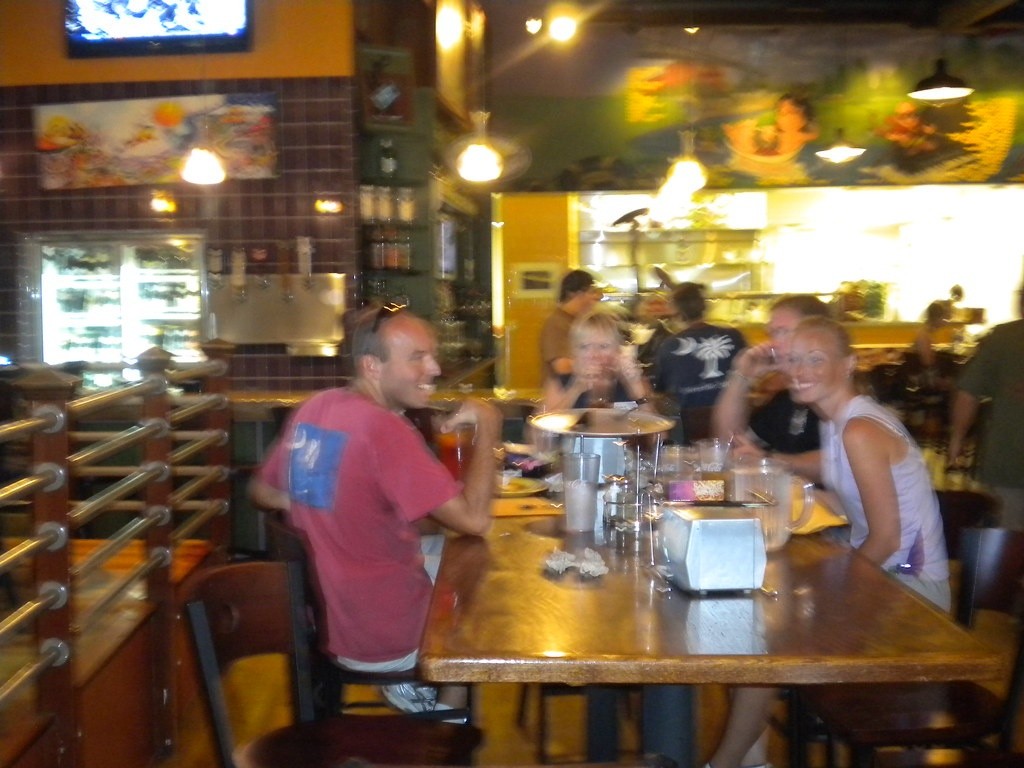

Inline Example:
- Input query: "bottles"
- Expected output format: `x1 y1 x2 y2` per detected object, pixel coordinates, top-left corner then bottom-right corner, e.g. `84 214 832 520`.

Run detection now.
357 180 417 274
604 475 622 517
378 137 398 180
617 478 637 523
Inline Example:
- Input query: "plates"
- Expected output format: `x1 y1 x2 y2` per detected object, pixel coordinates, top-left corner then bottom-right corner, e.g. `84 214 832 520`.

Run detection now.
497 477 551 498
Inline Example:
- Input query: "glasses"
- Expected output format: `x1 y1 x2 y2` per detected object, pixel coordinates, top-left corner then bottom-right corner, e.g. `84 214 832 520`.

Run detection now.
370 297 407 337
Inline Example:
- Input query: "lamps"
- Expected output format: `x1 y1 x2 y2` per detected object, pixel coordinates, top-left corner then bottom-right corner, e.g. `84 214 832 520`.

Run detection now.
907 11 973 102
813 127 869 166
665 27 707 194
181 29 226 185
455 17 506 181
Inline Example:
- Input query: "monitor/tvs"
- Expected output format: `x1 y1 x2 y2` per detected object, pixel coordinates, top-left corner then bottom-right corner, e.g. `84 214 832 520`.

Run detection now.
63 0 253 60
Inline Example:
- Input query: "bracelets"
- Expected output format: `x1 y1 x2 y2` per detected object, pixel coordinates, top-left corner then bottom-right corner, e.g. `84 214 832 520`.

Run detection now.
631 393 651 405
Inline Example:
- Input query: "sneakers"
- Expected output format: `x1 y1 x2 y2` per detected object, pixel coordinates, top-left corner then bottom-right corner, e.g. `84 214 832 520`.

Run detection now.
381 683 437 714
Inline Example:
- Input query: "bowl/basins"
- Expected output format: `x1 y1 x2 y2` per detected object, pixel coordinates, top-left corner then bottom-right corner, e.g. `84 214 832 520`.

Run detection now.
524 406 678 458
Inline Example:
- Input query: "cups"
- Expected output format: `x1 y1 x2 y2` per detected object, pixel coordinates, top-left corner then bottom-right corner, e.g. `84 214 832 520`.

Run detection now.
693 437 727 472
430 415 476 482
559 452 601 533
660 444 702 490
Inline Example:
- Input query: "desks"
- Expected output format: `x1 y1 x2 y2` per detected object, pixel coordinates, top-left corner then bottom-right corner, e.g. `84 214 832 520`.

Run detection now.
73 388 316 555
415 490 1004 768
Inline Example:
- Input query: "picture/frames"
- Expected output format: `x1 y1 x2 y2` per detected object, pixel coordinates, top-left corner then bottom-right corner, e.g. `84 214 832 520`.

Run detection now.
356 41 419 134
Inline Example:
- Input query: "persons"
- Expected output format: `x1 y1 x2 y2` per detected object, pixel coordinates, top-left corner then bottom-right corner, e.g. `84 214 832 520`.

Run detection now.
947 276 1024 626
525 269 953 768
251 303 502 726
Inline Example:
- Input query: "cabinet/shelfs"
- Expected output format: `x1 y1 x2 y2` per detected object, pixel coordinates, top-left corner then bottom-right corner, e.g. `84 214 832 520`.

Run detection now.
359 175 499 393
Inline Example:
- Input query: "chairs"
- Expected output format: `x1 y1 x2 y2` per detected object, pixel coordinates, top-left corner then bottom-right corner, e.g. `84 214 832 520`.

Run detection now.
872 349 950 438
680 402 717 443
769 492 1024 768
177 511 485 767
406 405 452 448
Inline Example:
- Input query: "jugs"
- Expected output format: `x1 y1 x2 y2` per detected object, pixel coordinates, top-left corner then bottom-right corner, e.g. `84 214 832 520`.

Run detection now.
730 456 816 554
